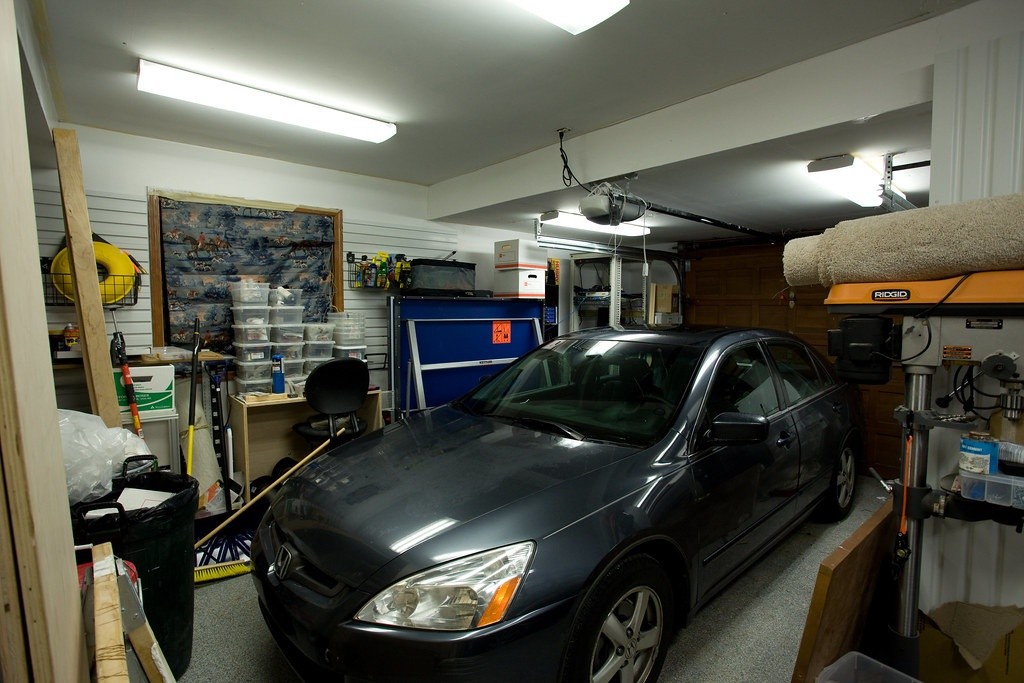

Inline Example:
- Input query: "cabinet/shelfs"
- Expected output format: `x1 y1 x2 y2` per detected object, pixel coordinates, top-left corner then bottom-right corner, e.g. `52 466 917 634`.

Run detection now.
569 252 681 333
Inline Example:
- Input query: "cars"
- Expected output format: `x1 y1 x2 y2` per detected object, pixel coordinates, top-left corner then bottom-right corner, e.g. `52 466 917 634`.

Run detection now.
245 322 864 683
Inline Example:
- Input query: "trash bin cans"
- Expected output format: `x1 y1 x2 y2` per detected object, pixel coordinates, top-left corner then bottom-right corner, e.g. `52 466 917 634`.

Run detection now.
70 455 201 683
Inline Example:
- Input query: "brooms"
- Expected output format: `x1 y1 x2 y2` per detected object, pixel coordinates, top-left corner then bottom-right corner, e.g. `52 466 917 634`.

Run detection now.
192 559 251 583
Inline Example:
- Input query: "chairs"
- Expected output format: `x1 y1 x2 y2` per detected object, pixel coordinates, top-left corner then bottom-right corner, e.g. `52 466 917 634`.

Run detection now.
603 357 666 403
706 356 756 416
292 358 370 455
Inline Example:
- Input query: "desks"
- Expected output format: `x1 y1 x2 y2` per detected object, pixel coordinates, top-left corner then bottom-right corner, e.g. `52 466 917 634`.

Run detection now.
227 386 383 506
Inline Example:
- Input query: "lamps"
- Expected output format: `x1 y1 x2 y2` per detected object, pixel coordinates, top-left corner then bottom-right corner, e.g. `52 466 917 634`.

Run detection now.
807 154 908 208
540 210 651 237
136 57 397 144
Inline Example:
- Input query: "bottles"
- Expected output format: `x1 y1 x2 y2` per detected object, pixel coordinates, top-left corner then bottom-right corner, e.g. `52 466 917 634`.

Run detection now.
67 322 73 329
360 255 367 265
381 269 386 287
110 332 127 368
366 263 377 287
272 354 284 393
48 330 66 364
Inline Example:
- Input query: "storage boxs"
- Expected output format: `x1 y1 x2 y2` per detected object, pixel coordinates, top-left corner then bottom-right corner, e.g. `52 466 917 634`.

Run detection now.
270 325 305 343
269 306 306 325
654 284 682 324
233 342 273 363
303 357 334 375
233 360 274 381
231 306 271 325
230 281 271 306
268 286 303 306
493 239 548 299
231 325 272 344
304 323 336 341
327 311 367 347
283 358 306 378
332 344 367 360
271 342 305 361
234 377 274 394
814 651 924 683
302 341 336 357
112 363 176 419
